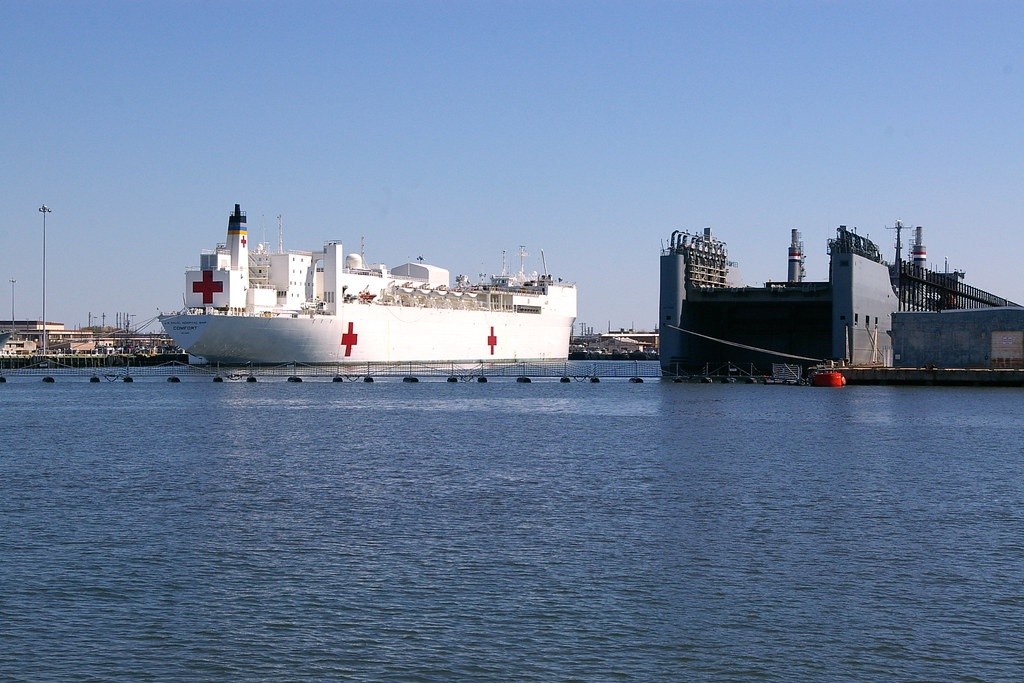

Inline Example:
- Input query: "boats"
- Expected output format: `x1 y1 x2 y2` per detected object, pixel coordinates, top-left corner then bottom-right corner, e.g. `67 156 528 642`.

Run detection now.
158 203 577 361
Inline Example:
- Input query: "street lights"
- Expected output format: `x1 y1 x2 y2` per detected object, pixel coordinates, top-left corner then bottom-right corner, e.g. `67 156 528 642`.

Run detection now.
39 204 51 354
8 278 17 330
